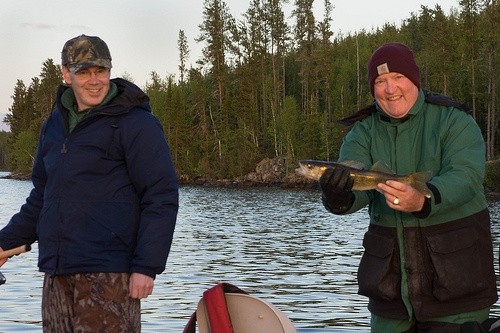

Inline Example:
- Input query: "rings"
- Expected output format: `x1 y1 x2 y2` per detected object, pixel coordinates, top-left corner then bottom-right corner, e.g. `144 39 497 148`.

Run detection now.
394 198 399 205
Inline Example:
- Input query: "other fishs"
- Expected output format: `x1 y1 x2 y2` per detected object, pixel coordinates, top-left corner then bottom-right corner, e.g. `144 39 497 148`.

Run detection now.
294 159 432 199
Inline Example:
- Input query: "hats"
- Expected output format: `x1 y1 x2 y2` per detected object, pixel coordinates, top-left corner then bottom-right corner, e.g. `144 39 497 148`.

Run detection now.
61 34 112 74
369 42 420 97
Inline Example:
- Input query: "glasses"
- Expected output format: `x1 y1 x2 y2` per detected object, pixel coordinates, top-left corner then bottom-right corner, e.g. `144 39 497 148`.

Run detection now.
74 68 109 80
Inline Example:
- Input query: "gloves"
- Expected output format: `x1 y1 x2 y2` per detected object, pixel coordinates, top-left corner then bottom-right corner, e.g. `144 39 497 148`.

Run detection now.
318 166 354 213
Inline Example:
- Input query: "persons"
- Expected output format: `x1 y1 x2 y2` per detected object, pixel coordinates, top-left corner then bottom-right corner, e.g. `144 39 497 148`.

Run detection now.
0 35 179 333
319 43 499 333
